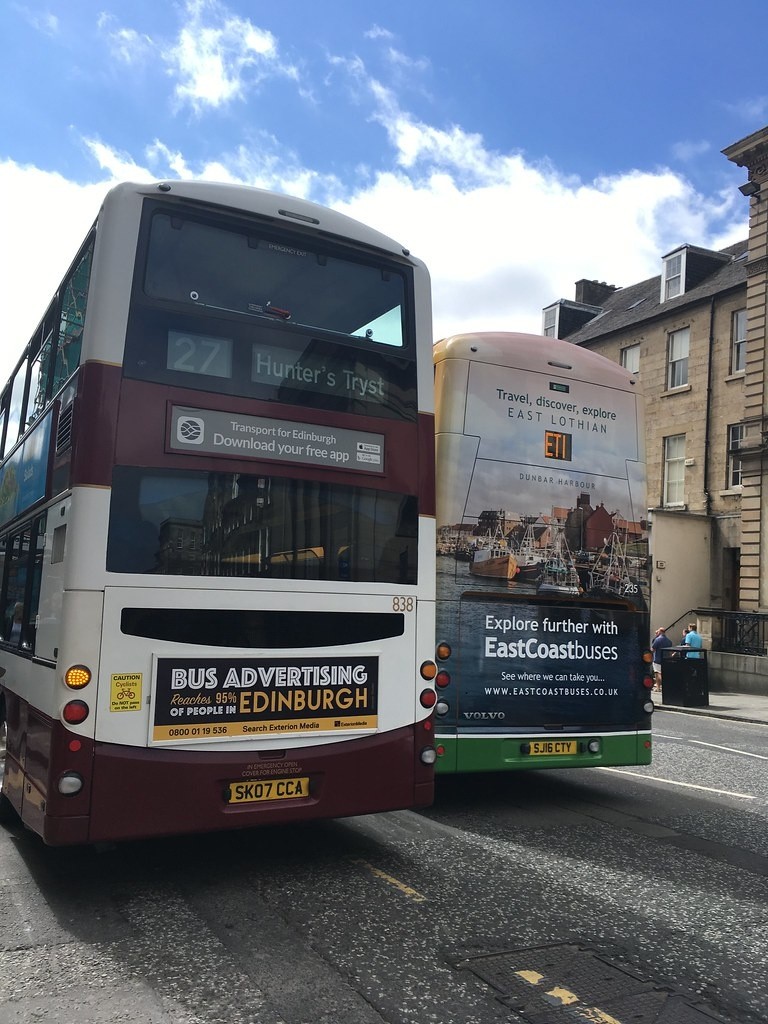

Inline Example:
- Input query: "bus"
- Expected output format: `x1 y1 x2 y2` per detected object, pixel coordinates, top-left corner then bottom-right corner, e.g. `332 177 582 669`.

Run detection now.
433 332 654 773
0 181 438 853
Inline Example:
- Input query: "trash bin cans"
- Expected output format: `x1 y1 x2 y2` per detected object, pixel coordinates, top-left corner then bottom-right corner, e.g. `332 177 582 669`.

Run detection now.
660 646 710 706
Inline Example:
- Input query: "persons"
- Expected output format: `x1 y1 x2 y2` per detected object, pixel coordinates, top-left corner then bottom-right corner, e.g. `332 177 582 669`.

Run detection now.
671 629 689 657
684 623 702 658
5 602 24 643
651 628 672 692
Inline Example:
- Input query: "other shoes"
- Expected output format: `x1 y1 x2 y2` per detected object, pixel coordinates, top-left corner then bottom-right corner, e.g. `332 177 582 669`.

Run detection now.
653 687 661 692
